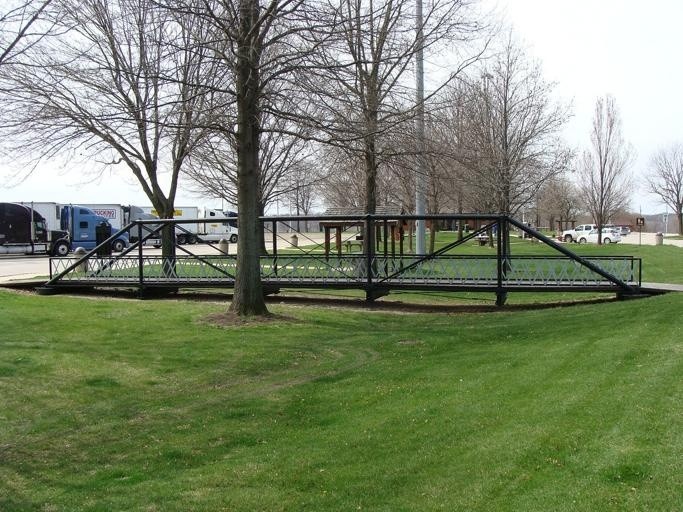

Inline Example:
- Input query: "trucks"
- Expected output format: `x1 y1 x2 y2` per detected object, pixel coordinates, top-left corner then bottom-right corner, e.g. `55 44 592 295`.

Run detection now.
0 202 238 257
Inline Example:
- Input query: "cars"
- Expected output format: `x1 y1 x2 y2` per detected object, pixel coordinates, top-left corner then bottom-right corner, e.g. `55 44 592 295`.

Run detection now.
561 224 631 245
